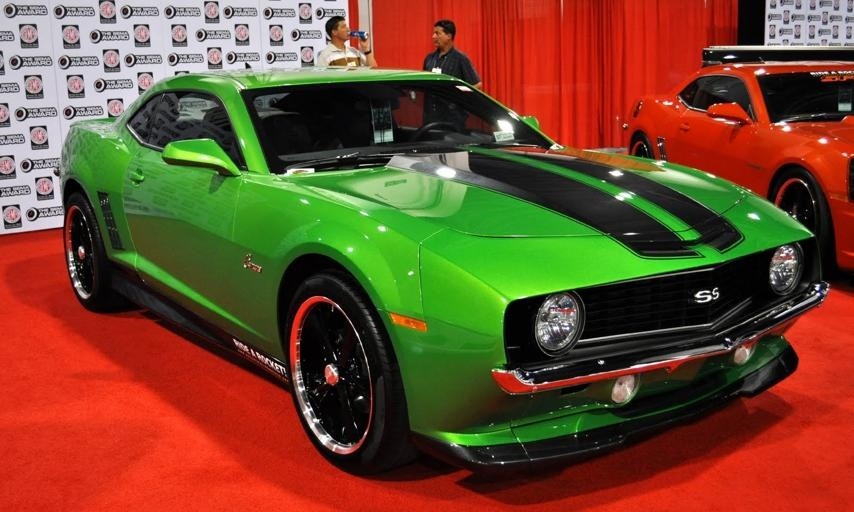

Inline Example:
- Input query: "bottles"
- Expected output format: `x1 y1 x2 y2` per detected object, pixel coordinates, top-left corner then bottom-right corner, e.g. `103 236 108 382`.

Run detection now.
347 31 368 41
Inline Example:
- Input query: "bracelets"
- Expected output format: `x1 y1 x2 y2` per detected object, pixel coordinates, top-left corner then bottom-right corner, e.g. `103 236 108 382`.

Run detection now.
364 51 371 55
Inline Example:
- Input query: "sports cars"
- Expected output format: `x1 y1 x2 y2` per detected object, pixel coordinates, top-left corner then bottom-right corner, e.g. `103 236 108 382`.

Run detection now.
622 54 854 285
56 59 833 484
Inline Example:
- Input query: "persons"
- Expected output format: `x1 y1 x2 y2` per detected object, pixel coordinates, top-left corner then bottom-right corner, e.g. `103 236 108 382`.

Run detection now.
317 15 378 68
423 20 483 128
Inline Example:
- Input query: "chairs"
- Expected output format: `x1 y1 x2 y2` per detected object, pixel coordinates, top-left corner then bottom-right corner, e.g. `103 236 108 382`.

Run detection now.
263 114 312 154
